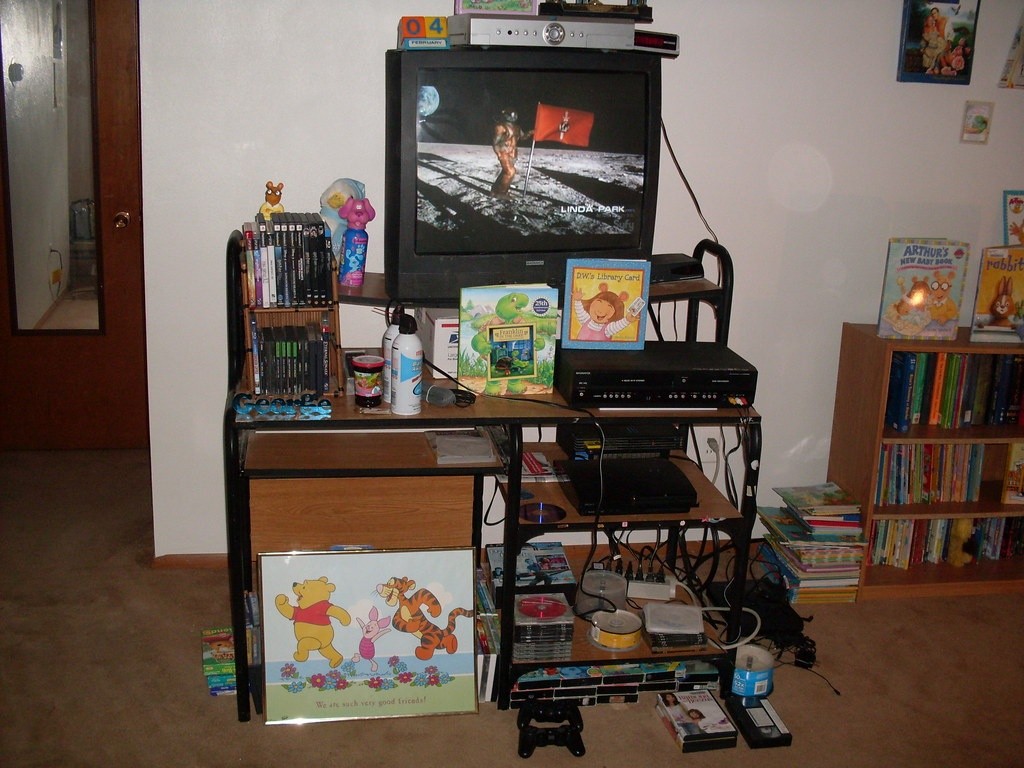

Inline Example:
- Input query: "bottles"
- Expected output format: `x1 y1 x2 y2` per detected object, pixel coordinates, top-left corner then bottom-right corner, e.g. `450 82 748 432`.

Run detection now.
382 304 423 416
337 198 376 288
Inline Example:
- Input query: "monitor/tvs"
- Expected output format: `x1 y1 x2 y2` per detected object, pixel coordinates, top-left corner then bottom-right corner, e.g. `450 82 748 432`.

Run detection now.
385 49 662 297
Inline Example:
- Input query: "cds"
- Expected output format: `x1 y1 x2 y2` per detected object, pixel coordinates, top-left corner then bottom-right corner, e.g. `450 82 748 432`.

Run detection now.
520 503 567 522
517 596 567 620
577 597 643 648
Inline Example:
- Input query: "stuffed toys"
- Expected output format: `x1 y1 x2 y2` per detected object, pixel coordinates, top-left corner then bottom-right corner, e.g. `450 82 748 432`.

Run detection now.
947 519 974 567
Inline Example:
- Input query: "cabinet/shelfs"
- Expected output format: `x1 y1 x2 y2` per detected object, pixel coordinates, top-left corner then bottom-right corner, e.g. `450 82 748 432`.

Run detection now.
223 230 763 723
826 322 1024 603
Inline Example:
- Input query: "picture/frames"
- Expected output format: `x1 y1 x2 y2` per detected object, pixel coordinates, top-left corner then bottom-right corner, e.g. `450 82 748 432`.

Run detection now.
897 0 981 85
257 546 478 726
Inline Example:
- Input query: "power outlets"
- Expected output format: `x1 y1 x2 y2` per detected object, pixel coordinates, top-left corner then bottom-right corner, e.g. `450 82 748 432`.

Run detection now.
48 244 52 258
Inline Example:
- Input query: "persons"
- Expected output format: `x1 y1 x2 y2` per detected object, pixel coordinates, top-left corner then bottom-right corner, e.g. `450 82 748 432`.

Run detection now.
492 567 503 579
524 558 552 585
661 693 700 736
490 107 536 198
920 7 955 73
688 709 735 733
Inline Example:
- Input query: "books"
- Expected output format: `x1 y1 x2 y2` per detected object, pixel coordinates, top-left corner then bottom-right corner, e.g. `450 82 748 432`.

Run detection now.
867 519 1024 570
1003 189 1024 247
561 259 651 350
425 429 496 464
201 626 237 695
1001 441 1024 504
457 284 559 394
496 452 570 484
877 237 969 340
755 482 868 604
874 443 985 507
969 244 1024 345
885 351 1024 434
